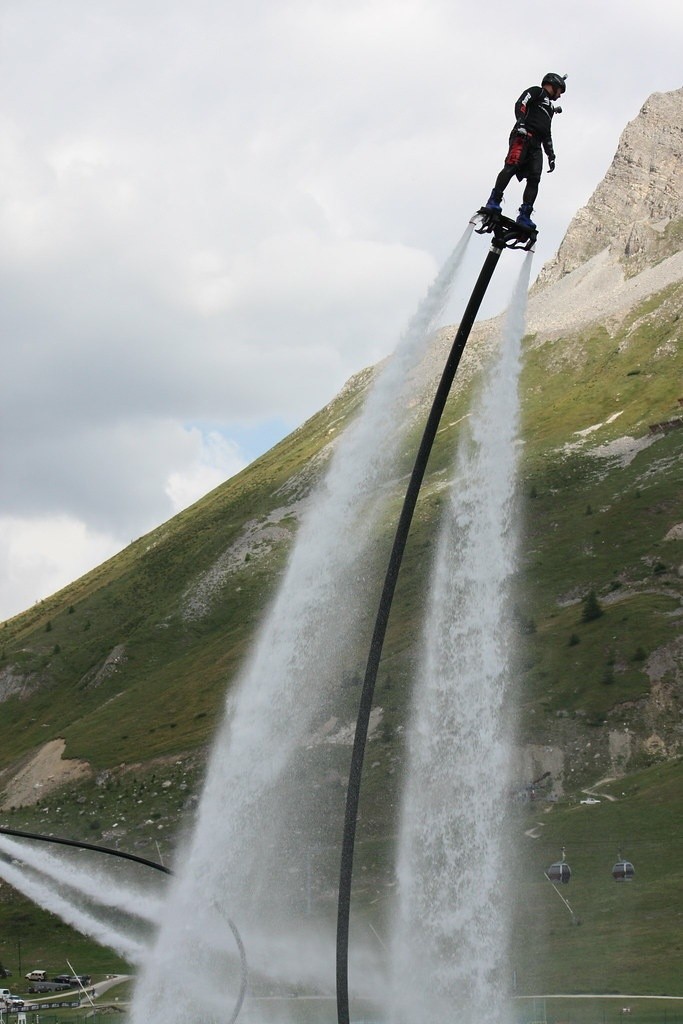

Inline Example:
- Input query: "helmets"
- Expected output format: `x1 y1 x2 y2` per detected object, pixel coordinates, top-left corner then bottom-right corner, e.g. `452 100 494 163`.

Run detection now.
542 72 567 94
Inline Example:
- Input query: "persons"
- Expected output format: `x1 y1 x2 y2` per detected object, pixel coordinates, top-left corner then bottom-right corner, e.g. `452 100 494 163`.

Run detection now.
484 73 568 229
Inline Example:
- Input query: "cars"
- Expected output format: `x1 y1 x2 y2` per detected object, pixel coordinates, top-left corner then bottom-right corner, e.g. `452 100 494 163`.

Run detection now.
4 969 13 977
69 976 86 986
50 975 72 984
55 984 72 991
4 995 24 1008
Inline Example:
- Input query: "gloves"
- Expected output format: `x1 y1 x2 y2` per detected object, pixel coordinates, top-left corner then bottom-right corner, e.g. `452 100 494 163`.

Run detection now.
518 124 528 142
547 155 556 173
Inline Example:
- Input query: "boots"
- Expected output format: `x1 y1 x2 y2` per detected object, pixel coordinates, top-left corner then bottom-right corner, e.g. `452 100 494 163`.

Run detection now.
516 204 538 229
487 188 504 213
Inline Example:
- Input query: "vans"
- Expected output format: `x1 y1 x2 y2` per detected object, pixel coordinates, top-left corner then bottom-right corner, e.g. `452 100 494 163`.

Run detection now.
0 989 11 1002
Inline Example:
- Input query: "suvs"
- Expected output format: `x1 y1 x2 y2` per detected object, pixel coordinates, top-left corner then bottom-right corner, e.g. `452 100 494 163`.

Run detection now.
25 970 47 982
28 985 52 994
